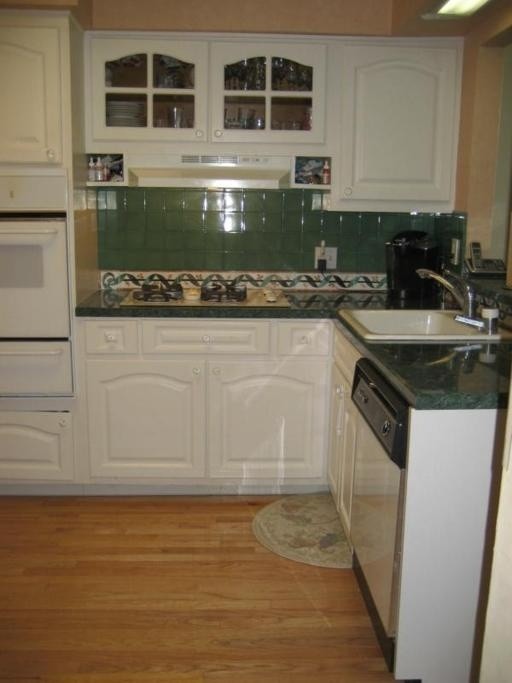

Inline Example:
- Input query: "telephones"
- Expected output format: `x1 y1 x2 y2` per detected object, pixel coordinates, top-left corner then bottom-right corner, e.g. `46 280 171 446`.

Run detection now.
466 242 507 277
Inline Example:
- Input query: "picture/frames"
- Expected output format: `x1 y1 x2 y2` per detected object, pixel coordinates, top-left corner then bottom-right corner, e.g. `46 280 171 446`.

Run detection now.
84 149 129 186
290 153 335 190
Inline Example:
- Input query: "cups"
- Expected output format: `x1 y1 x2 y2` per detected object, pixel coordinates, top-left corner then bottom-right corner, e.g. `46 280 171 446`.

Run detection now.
237 106 256 129
167 106 185 127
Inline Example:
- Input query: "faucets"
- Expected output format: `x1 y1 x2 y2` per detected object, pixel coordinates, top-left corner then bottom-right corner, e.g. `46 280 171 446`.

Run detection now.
420 346 471 371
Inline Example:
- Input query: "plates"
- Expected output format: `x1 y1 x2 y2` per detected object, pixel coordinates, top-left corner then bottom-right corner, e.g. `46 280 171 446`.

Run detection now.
105 102 146 127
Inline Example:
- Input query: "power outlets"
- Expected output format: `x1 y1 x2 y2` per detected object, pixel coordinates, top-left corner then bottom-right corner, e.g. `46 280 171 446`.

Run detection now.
449 238 459 265
314 246 337 270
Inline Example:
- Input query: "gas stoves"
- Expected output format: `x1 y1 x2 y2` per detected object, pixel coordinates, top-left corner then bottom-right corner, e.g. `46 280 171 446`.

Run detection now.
119 283 292 306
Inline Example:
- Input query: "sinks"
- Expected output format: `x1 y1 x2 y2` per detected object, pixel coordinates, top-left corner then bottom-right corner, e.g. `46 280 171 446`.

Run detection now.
338 309 512 342
416 267 475 319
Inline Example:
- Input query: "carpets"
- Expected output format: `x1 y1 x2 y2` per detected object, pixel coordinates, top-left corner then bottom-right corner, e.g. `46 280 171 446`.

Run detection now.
252 495 354 568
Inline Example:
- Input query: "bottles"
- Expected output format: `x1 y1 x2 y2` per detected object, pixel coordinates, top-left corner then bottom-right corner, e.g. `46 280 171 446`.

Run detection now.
88 156 108 182
301 111 310 130
323 161 330 184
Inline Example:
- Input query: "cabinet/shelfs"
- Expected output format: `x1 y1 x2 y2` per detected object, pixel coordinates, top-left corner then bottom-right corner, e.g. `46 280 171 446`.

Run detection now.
90 38 327 144
0 411 74 481
86 320 331 479
329 328 363 535
0 340 74 397
326 44 458 202
0 26 85 163
